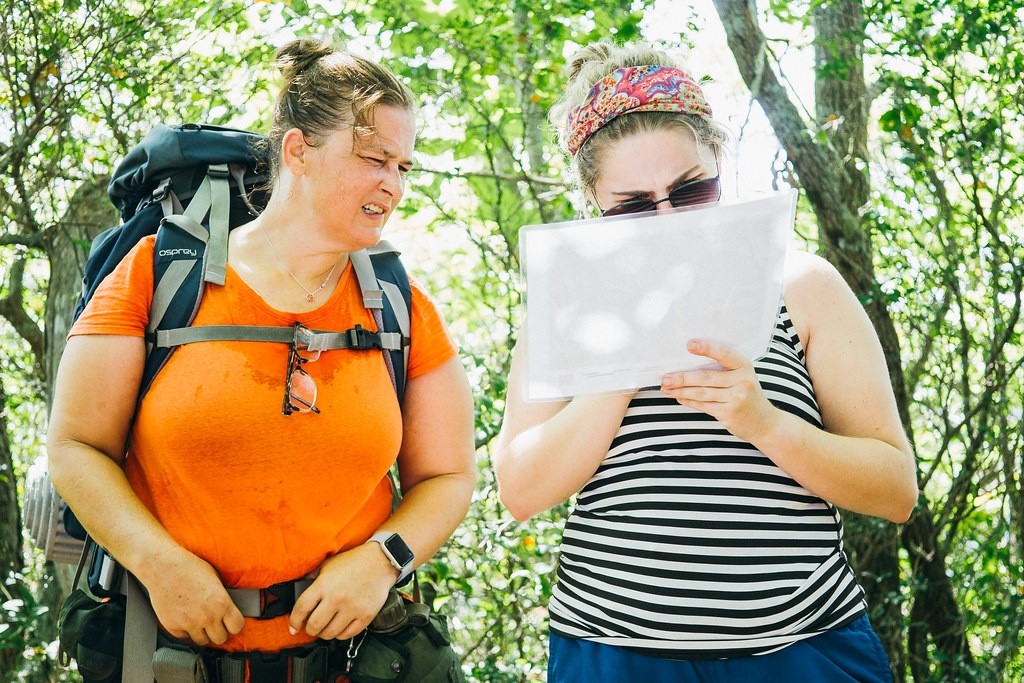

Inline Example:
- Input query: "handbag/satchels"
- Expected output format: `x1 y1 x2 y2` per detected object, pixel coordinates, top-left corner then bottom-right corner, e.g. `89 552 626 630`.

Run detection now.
58 588 124 682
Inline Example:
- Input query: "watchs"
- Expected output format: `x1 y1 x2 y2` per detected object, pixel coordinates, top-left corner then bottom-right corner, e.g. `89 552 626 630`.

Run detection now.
366 533 415 585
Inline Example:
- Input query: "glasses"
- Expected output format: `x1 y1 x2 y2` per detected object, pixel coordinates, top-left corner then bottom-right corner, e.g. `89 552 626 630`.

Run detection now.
280 321 321 416
589 145 721 217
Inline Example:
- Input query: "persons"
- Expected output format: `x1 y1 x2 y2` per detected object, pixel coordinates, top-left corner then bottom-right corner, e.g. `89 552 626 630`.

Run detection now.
46 39 476 683
496 42 918 683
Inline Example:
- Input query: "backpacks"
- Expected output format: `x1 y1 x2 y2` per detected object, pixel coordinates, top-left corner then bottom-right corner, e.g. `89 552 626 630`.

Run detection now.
64 123 411 540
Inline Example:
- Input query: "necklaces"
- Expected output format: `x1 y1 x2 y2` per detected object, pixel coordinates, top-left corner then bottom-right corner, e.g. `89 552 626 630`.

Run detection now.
258 222 335 301
